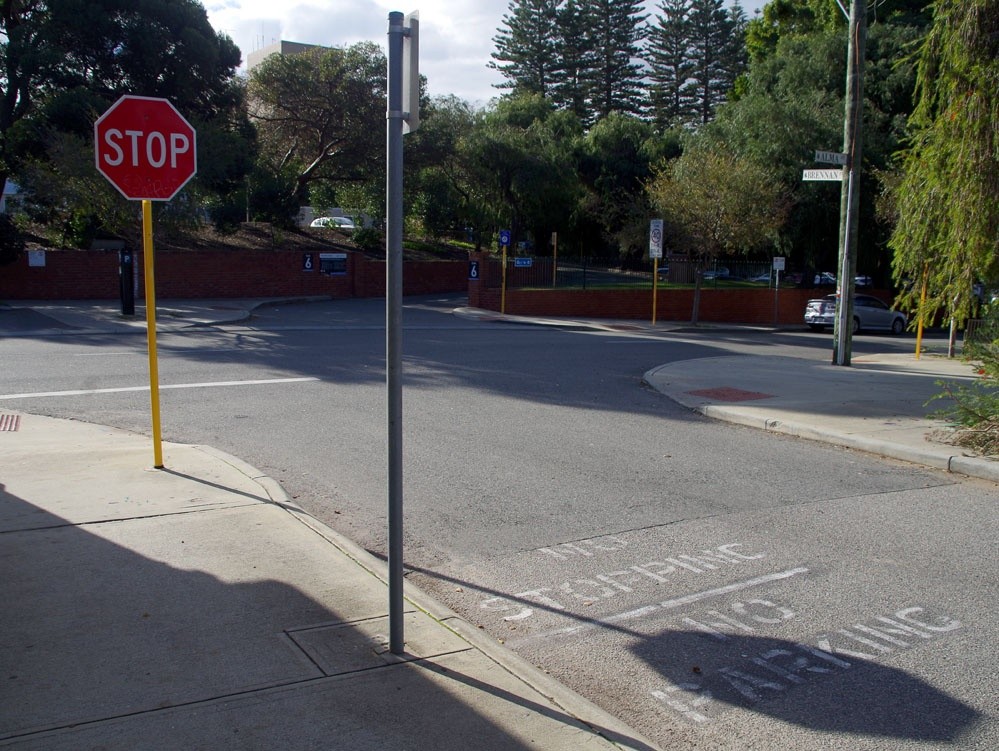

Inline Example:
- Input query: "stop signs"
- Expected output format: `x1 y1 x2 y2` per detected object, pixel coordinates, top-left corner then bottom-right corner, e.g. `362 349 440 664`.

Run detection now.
95 95 197 200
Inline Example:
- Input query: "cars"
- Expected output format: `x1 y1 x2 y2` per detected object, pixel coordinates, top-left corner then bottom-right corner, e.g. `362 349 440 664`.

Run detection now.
310 216 361 229
804 293 908 336
703 270 744 281
784 270 872 286
755 273 774 283
